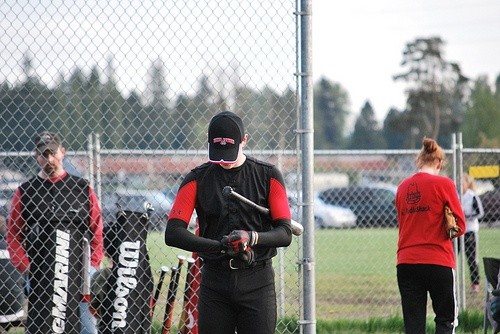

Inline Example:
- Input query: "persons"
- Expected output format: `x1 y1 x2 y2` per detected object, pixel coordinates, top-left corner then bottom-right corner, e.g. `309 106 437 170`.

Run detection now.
395 138 466 334
6 131 103 334
0 198 31 334
452 172 485 293
165 112 293 334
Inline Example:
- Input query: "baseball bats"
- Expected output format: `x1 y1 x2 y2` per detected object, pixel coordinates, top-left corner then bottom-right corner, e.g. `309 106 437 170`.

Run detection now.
178 257 196 334
161 255 186 334
152 266 169 316
222 186 304 236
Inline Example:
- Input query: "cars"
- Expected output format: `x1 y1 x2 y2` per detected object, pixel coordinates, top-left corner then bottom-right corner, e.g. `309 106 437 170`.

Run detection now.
0 184 500 232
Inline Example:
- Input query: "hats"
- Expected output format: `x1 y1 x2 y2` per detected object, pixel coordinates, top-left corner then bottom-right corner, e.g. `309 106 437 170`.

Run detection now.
34 132 61 156
207 111 245 165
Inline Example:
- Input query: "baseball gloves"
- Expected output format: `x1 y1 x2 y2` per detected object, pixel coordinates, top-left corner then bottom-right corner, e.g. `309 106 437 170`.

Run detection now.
444 205 462 240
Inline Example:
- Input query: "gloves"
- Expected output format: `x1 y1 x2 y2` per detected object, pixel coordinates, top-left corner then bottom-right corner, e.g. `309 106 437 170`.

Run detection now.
220 230 256 266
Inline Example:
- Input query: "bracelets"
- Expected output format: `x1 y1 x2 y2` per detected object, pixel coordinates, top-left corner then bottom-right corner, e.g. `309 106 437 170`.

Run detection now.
250 230 259 247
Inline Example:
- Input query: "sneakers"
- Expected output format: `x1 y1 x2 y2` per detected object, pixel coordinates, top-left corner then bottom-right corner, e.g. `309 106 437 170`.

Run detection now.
471 284 479 293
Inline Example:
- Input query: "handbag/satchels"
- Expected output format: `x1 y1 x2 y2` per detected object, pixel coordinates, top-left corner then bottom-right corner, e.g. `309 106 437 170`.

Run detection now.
445 207 458 237
